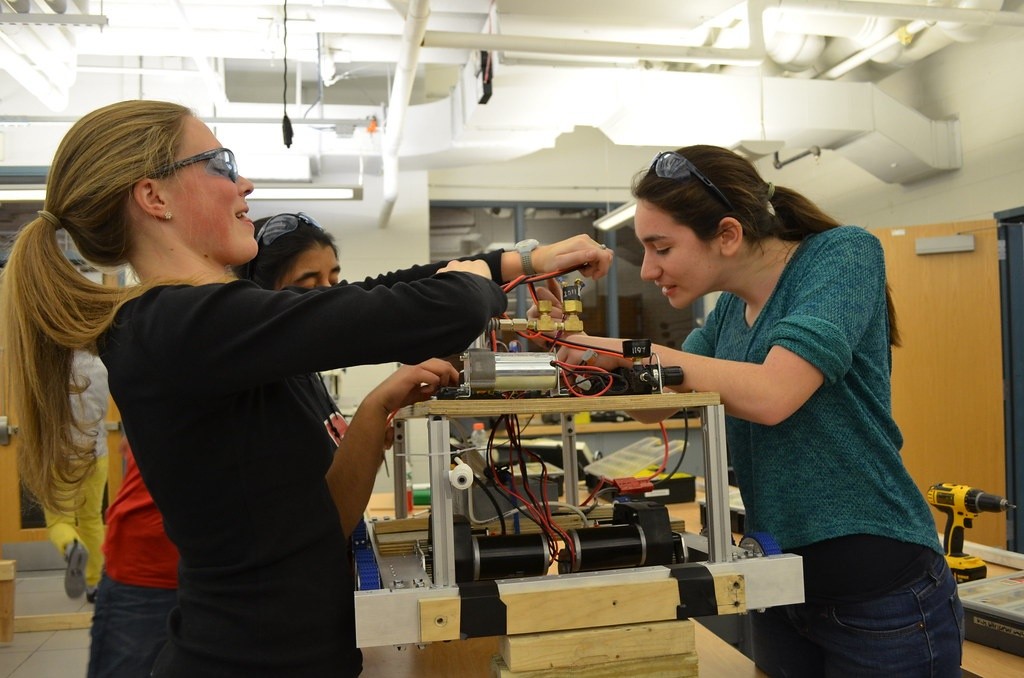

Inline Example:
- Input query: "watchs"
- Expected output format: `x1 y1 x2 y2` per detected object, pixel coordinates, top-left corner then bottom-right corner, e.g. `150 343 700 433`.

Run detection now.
515 239 539 275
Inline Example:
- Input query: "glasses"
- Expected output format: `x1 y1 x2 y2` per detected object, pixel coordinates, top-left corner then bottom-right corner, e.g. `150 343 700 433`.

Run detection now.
645 151 746 236
246 211 321 280
131 148 238 194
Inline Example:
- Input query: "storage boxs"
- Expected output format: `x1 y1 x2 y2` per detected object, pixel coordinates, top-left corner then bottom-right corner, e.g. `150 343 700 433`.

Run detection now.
957 570 1024 658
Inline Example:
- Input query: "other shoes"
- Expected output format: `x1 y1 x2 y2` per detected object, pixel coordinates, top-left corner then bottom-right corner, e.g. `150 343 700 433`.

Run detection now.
64 539 87 600
85 584 100 603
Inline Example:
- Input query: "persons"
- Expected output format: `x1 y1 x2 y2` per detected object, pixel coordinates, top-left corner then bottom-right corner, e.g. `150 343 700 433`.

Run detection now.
43 350 110 604
86 215 460 678
526 145 965 678
0 99 614 678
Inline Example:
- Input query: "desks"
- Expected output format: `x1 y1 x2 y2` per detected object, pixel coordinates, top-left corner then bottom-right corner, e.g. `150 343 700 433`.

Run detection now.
360 492 767 678
559 475 1024 678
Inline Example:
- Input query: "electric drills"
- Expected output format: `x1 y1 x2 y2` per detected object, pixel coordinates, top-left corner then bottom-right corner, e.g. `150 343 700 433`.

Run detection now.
927 481 1016 585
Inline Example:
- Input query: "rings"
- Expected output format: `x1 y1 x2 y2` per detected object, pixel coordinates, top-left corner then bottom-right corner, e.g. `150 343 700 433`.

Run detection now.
600 244 606 250
